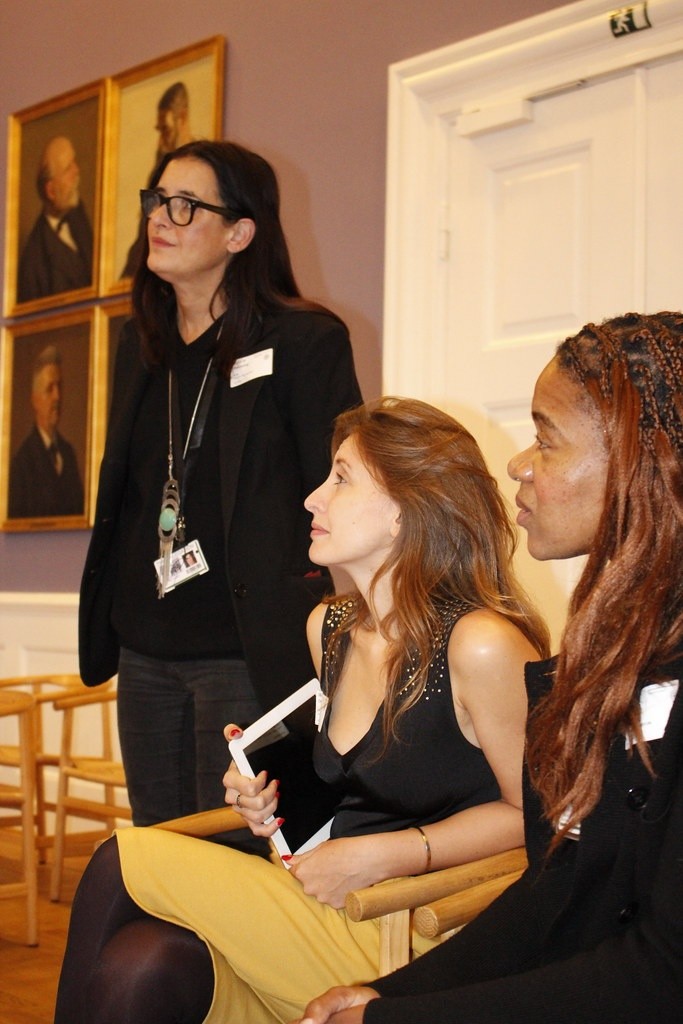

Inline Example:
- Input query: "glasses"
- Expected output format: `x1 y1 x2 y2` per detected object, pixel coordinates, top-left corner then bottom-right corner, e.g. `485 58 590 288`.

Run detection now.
140 189 241 226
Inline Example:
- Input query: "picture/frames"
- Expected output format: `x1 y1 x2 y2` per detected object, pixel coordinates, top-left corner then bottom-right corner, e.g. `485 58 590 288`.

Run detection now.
92 300 136 528
1 305 96 531
3 75 105 321
99 33 228 299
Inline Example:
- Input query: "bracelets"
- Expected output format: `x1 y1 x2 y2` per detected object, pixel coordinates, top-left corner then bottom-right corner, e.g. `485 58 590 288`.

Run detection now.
410 823 432 876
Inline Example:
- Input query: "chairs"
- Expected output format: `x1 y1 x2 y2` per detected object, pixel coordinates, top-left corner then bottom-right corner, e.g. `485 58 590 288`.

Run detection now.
0 675 531 1024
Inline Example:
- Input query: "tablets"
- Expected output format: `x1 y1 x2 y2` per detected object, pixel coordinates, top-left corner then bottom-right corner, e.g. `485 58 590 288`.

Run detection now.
227 678 343 871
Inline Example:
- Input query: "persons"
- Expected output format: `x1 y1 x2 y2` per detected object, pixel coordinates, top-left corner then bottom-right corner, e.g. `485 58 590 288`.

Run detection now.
77 138 365 823
9 345 83 519
15 136 91 301
294 311 683 1024
53 398 550 1023
120 81 190 278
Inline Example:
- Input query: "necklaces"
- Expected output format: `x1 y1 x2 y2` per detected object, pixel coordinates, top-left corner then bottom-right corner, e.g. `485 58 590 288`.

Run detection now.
155 314 226 603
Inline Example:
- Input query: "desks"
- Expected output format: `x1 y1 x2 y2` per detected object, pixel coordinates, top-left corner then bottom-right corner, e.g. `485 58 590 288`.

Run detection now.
0 690 42 946
